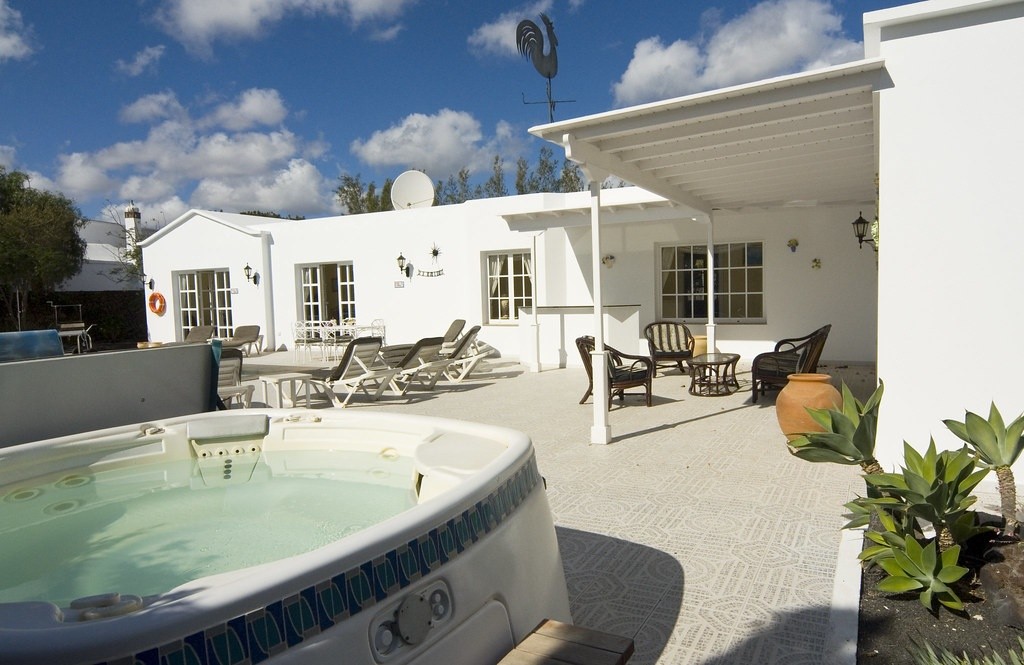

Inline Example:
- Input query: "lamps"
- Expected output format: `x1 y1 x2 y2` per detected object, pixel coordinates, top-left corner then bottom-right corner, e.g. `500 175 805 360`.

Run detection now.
396 252 409 278
244 262 257 285
852 210 874 250
140 273 154 289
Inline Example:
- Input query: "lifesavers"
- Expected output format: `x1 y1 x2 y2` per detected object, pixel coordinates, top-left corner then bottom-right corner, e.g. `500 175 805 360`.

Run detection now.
149 293 164 313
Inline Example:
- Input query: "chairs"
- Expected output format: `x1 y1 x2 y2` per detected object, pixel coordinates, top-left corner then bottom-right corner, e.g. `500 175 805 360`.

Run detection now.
379 325 495 385
644 321 695 379
221 325 260 357
575 336 653 412
160 325 214 347
752 323 832 404
46 300 98 355
295 335 403 411
217 348 256 409
293 317 386 366
444 318 466 341
372 336 444 399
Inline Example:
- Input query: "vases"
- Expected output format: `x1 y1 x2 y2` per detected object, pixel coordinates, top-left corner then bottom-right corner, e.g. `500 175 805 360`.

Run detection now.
137 342 148 348
605 261 613 267
776 372 843 442
791 246 796 252
150 341 161 347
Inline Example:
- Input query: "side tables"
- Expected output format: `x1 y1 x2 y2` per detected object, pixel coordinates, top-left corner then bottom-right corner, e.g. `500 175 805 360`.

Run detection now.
687 352 741 396
258 373 311 409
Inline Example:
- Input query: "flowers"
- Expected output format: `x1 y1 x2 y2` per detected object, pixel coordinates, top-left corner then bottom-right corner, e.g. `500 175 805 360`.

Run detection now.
787 239 799 247
602 254 614 264
